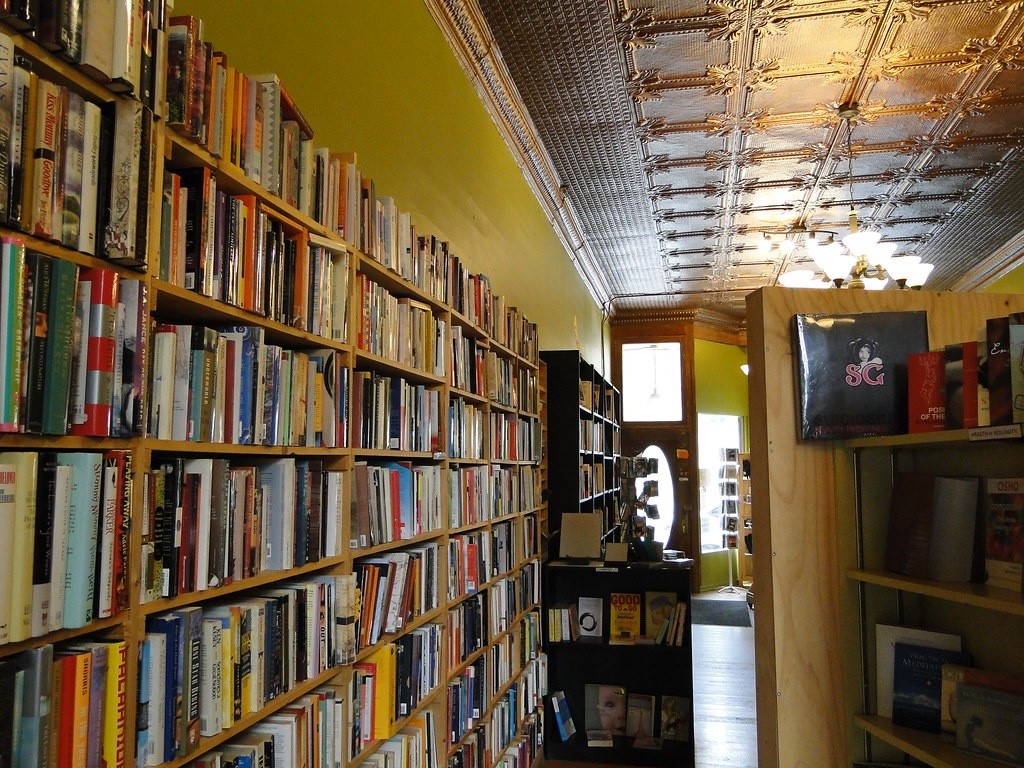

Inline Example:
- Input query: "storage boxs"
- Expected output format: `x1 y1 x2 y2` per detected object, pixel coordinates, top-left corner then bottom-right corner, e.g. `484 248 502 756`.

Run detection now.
792 310 929 442
908 311 1024 434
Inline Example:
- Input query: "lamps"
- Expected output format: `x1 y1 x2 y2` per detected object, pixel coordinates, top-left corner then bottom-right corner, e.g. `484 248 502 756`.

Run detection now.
757 109 935 290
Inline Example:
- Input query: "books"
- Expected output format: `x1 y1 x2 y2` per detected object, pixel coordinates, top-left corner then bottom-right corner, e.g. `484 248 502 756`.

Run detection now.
0 0 692 768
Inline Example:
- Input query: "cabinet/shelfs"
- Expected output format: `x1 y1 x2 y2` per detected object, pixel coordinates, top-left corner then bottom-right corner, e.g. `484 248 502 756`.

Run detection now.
0 0 694 768
844 423 1024 768
715 448 754 595
